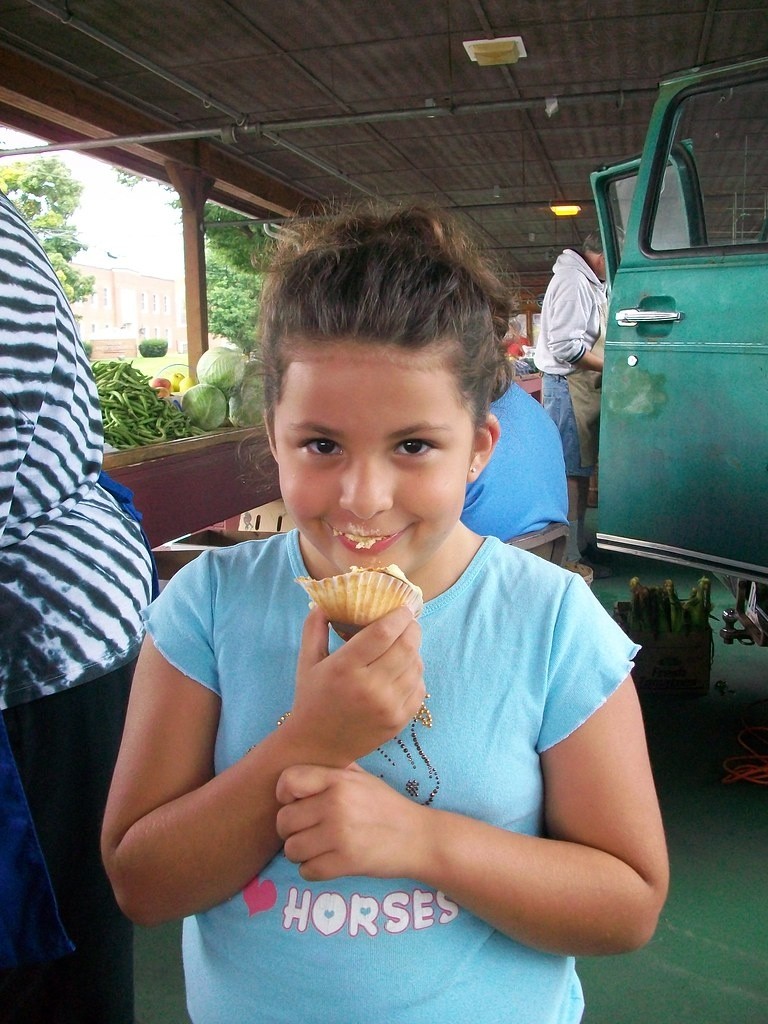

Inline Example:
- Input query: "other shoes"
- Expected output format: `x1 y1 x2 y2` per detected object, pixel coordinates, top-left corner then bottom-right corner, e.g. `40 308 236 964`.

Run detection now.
565 563 595 584
580 544 607 565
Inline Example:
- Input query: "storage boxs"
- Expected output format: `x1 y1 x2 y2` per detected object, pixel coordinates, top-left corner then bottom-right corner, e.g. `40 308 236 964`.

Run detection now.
612 597 713 698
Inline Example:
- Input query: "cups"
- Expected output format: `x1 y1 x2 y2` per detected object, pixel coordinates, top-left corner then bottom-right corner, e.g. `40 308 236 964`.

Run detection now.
166 395 181 410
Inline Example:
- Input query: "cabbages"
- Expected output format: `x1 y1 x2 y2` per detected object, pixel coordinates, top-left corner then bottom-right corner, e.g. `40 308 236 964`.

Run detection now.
183 346 272 431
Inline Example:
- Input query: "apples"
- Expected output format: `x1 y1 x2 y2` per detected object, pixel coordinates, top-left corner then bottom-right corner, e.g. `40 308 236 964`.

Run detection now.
179 377 197 393
168 373 185 392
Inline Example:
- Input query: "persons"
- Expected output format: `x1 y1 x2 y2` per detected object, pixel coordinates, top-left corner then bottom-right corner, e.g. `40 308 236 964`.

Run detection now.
459 316 571 543
101 203 669 1024
533 226 625 578
0 189 160 1024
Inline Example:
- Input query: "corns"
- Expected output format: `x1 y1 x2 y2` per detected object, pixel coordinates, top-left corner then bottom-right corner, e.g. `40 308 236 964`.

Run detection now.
625 576 712 645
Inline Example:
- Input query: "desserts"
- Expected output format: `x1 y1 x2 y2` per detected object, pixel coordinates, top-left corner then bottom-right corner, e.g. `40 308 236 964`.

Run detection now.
295 564 423 641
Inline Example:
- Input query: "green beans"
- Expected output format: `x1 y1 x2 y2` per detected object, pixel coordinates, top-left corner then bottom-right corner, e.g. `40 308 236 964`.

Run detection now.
93 359 207 450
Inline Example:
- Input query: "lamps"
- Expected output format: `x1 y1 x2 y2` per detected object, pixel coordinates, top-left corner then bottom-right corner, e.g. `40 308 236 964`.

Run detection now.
460 35 528 67
547 200 583 215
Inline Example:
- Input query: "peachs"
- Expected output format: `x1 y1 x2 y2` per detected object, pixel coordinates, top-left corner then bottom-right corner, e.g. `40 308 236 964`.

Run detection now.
150 378 171 390
154 387 170 399
